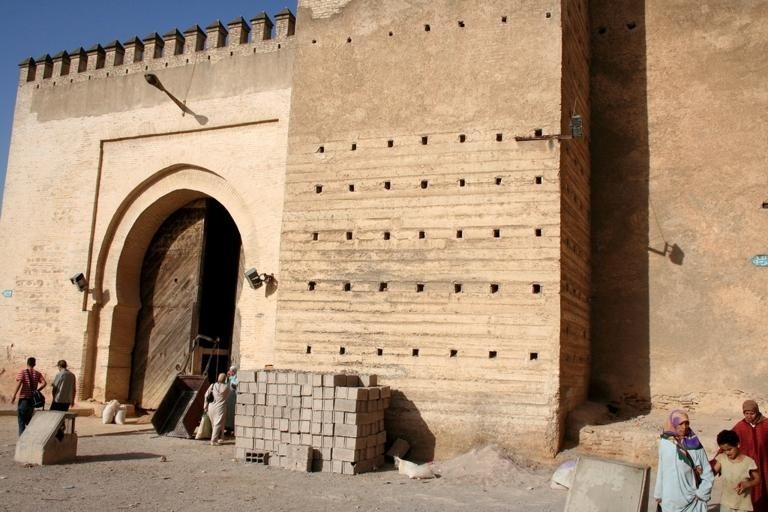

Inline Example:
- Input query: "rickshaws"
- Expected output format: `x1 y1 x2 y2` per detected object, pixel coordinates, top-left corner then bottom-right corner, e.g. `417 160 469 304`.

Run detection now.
150 335 220 440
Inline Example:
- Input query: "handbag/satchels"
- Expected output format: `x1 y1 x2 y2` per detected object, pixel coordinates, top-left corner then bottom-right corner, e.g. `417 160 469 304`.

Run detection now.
195 410 212 440
207 383 213 402
32 390 45 407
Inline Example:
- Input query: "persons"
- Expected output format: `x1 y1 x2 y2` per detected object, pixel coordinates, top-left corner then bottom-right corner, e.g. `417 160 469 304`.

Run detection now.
49 358 77 410
695 430 762 512
722 398 768 512
10 356 47 435
653 410 714 512
225 365 239 390
203 372 229 446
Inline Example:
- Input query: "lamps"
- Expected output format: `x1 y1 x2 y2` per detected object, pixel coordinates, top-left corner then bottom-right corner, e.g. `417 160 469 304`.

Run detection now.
515 115 584 142
70 272 88 292
245 268 273 290
